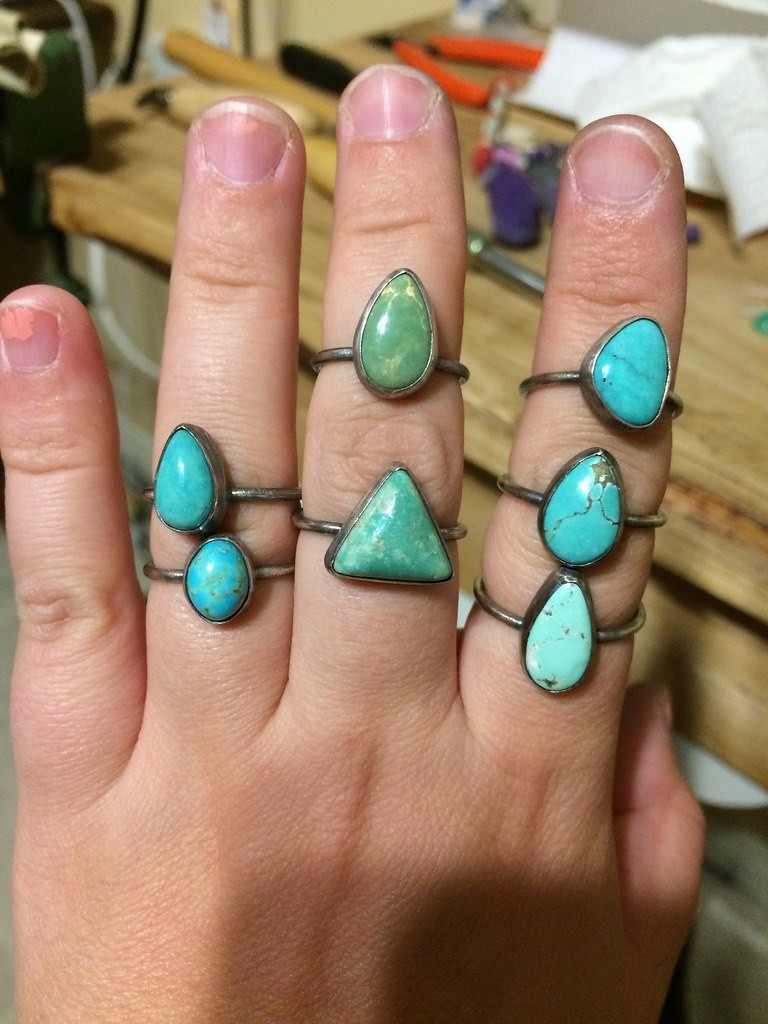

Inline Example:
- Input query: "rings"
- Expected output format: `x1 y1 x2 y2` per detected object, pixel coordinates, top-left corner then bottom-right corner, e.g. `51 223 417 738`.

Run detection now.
474 567 646 694
312 266 469 400
144 536 296 626
144 422 300 534
496 447 668 569
519 316 684 432
292 460 467 587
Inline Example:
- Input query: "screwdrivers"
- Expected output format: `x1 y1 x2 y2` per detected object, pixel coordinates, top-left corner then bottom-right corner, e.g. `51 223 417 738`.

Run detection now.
307 137 551 300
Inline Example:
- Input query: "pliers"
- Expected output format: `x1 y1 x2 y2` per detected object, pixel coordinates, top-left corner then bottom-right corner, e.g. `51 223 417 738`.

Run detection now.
364 30 544 110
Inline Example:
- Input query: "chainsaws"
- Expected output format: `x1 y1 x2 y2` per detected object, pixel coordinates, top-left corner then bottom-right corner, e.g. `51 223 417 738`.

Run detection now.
471 78 700 248
